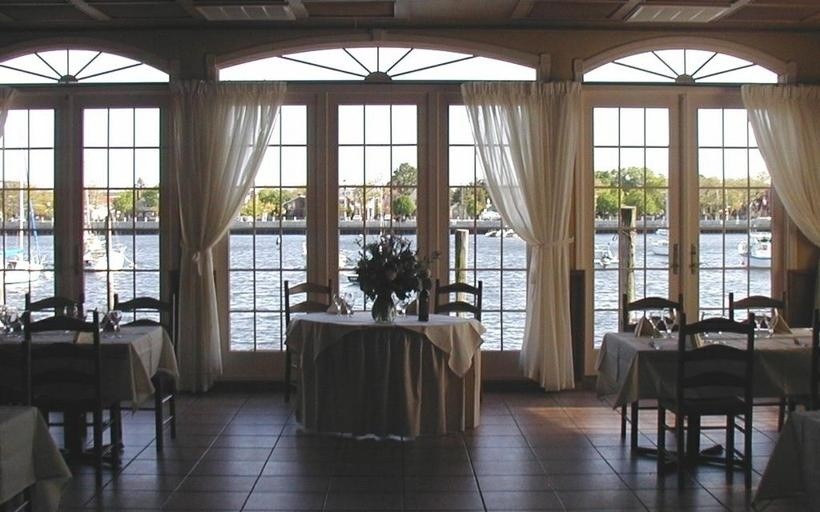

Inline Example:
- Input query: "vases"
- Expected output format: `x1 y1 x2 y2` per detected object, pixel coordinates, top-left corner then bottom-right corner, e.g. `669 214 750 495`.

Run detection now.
372 291 398 324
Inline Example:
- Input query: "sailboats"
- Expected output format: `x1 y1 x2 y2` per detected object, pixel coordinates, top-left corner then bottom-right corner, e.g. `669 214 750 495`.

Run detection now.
4 180 42 283
85 184 124 278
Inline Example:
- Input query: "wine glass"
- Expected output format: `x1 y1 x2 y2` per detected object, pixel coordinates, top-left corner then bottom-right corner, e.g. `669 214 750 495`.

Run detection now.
751 310 778 339
65 306 78 335
106 311 123 339
332 292 353 320
648 305 678 341
0 304 17 338
399 298 409 321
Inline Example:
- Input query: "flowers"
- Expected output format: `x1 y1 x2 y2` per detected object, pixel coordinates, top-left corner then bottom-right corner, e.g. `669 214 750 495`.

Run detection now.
348 230 440 301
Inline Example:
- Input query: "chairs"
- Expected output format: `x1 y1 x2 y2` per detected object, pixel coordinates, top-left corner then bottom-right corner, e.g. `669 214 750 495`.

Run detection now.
620 291 686 458
96 290 180 456
284 279 335 403
25 291 87 449
428 281 486 402
728 290 794 434
657 313 757 482
789 303 820 416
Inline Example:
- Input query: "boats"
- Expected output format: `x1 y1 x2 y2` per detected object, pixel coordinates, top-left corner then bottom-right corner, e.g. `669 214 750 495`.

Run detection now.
649 227 669 256
738 217 772 268
594 243 619 269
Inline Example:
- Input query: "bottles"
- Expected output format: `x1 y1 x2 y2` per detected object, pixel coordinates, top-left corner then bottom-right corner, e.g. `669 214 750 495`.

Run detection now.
419 288 429 322
